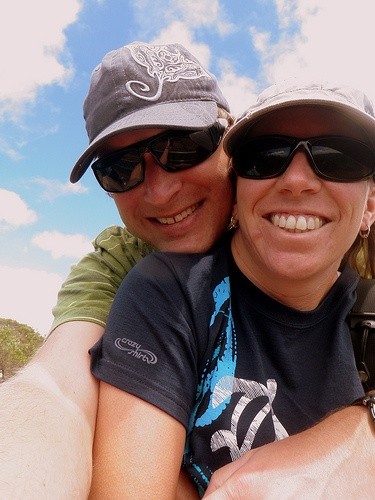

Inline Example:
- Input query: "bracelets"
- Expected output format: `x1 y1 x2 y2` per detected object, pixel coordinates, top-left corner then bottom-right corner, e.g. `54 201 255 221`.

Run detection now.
351 395 375 421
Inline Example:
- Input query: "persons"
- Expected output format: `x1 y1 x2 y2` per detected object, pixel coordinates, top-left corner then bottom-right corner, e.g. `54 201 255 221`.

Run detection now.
0 40 374 500
88 77 374 500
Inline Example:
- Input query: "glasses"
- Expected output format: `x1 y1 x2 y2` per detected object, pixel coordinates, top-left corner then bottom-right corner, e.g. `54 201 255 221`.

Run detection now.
232 133 375 183
91 118 229 193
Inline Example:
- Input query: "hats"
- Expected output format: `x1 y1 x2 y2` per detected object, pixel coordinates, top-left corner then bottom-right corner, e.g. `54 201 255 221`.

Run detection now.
70 41 230 183
222 79 375 159
